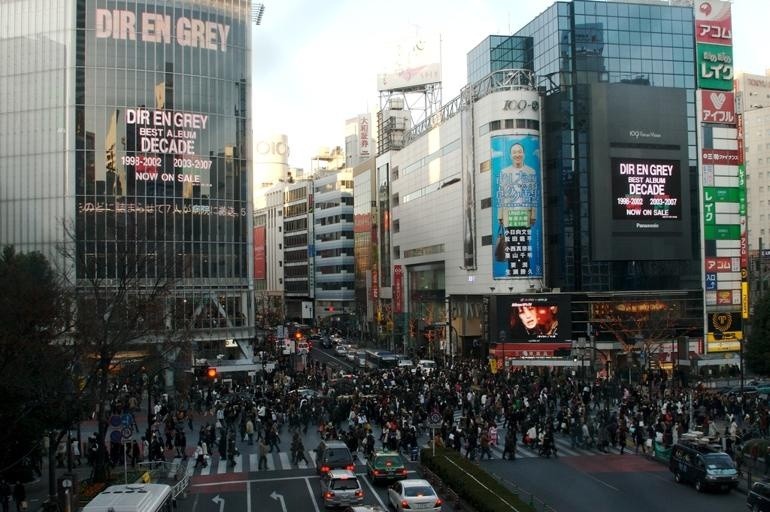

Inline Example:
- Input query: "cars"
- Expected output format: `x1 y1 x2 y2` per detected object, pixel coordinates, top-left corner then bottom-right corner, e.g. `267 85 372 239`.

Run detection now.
720 378 770 399
745 478 770 512
387 478 442 512
275 321 437 375
344 504 389 512
364 448 408 485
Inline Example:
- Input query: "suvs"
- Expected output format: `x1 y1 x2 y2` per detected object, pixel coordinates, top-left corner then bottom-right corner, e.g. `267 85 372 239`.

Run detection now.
313 440 358 476
319 468 363 509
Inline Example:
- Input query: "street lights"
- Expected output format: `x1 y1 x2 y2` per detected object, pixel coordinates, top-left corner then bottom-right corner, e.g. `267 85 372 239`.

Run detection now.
445 321 459 359
570 346 611 417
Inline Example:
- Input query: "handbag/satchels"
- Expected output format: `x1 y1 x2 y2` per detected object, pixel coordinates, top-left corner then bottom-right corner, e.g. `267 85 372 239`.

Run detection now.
494 230 507 263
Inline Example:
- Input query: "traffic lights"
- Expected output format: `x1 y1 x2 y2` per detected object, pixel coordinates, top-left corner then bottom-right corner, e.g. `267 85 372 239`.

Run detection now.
194 366 217 379
325 307 335 311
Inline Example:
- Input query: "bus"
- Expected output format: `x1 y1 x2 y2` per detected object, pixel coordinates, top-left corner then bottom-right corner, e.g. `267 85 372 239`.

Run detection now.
82 482 178 512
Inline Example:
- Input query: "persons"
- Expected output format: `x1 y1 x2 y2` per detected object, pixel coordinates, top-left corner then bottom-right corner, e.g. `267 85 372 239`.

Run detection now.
512 307 541 334
498 142 538 276
0 479 13 512
67 316 770 470
13 480 27 511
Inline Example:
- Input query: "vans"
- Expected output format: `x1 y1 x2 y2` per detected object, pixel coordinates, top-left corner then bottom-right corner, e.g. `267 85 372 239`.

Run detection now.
668 440 740 493
